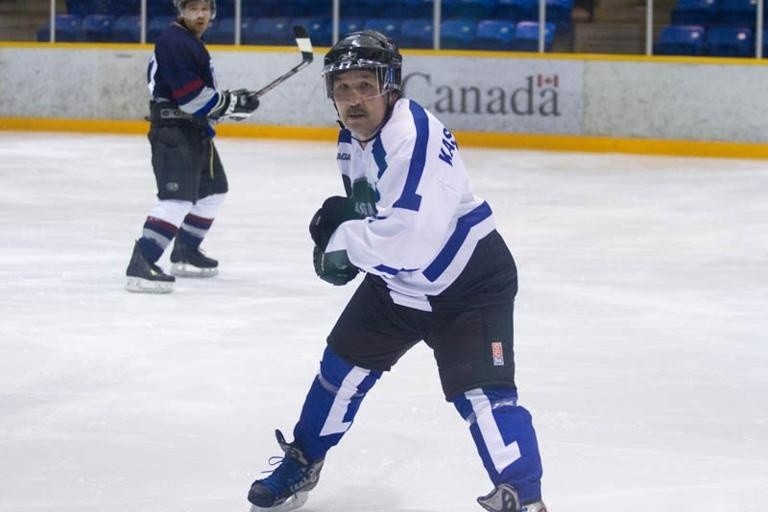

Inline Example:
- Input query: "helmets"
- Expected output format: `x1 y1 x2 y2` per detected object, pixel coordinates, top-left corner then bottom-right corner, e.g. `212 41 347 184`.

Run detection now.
172 0 217 23
324 29 403 93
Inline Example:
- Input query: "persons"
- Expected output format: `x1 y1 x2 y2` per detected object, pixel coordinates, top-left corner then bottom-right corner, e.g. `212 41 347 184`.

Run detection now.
124 0 259 283
246 32 548 512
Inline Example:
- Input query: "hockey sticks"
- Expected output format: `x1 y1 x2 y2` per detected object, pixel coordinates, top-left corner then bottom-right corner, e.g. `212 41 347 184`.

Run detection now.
253 25 313 98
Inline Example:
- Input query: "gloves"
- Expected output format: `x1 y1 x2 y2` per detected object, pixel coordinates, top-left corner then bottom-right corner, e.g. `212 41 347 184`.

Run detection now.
310 196 362 285
223 89 259 121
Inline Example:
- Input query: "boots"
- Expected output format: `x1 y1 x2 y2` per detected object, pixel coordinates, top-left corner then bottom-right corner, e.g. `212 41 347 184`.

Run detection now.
477 483 546 512
127 239 174 282
170 234 218 269
249 430 323 507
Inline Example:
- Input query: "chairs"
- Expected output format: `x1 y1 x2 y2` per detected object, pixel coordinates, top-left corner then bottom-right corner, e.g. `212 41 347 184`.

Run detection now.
654 0 768 58
